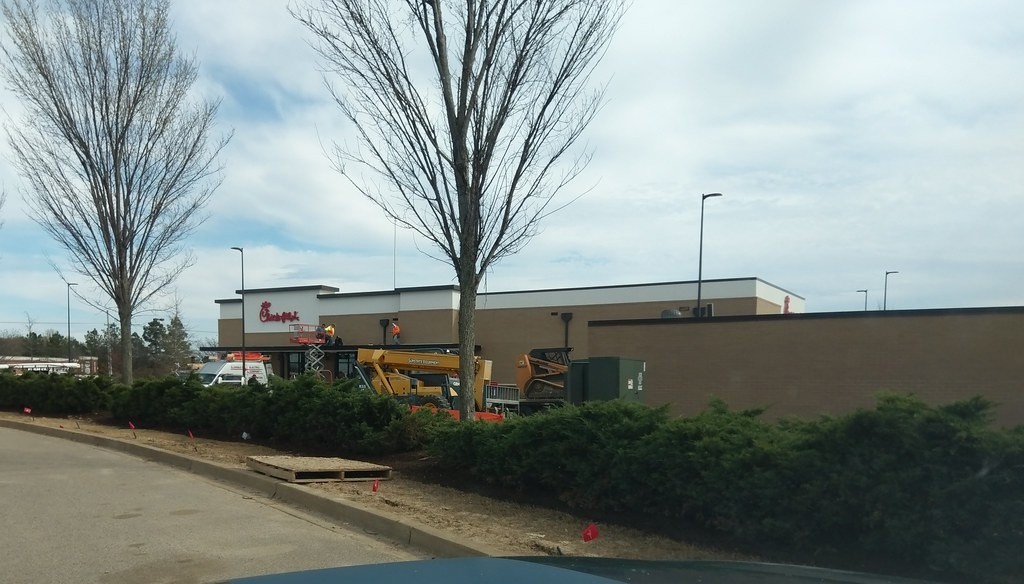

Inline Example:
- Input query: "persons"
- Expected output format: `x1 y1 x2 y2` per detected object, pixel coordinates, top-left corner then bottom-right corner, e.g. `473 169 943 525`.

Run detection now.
248 374 258 385
316 324 335 346
391 322 400 345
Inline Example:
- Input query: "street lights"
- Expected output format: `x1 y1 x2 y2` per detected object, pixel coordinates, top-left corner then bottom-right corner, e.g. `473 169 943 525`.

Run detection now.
856 290 867 311
884 271 900 310
231 246 247 376
68 282 78 362
697 193 723 316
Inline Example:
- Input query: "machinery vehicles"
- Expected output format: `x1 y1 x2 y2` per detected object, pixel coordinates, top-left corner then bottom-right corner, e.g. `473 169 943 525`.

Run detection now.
355 346 570 417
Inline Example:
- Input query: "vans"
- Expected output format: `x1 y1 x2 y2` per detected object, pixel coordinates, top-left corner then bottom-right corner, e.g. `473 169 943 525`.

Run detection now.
195 361 268 390
